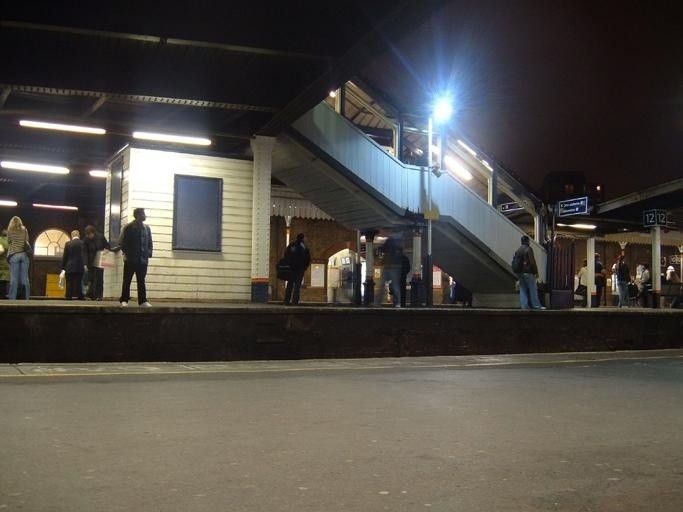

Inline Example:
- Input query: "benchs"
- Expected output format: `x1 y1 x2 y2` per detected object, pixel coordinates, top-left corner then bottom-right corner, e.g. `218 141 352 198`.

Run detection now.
627 278 682 308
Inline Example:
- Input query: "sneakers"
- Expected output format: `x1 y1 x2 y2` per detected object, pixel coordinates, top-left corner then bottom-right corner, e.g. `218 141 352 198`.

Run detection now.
119 301 129 310
137 301 153 308
534 306 546 310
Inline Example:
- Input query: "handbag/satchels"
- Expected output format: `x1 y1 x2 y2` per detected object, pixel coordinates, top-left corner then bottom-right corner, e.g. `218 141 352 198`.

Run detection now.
22 239 34 258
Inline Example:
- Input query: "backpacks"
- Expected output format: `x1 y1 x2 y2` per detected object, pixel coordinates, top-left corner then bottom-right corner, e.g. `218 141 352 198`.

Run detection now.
510 250 524 274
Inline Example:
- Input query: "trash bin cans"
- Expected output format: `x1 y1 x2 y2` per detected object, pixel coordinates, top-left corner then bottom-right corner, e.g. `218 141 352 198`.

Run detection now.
250 277 270 305
645 290 660 309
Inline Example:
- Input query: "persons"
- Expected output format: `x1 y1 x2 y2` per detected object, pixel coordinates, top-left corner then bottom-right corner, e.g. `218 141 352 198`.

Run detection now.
414 143 438 167
577 252 651 306
81 224 116 301
400 248 409 307
283 233 310 306
0 224 11 298
440 271 453 303
3 216 33 300
368 236 402 307
668 266 681 307
510 235 546 309
402 147 416 164
57 229 86 300
117 208 152 308
